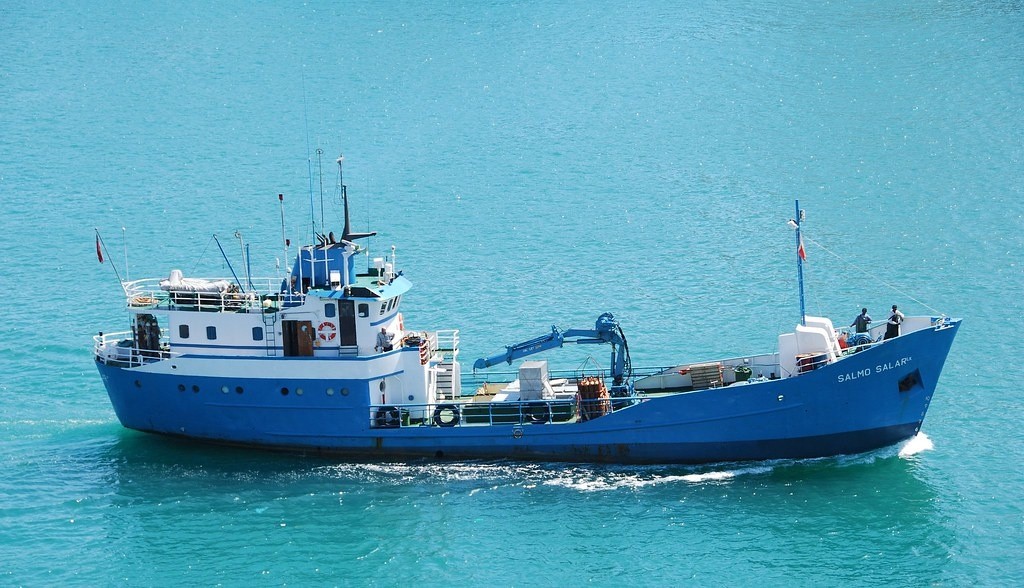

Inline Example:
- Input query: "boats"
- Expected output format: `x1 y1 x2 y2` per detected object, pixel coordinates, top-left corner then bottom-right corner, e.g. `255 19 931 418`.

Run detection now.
91 144 964 466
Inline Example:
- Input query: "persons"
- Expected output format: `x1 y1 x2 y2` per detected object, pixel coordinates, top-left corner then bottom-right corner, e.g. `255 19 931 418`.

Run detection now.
888 305 904 336
849 306 872 333
838 331 850 348
374 326 395 352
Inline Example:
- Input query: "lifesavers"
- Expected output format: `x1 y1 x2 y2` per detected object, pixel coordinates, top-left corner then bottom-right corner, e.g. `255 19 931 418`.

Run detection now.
377 407 400 428
317 322 336 340
525 402 550 425
434 405 459 427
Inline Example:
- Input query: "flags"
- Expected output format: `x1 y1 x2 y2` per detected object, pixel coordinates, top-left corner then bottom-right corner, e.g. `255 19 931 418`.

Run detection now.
96 234 105 264
799 237 806 261
